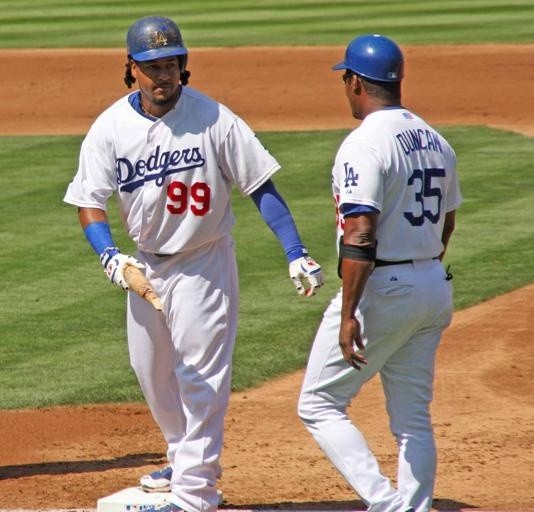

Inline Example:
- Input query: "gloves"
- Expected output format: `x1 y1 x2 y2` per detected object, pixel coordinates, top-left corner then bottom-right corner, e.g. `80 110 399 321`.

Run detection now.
99 247 145 292
289 255 324 296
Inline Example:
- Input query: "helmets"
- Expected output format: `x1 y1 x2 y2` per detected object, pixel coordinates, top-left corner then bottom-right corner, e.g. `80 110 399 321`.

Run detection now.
331 35 403 82
126 16 187 61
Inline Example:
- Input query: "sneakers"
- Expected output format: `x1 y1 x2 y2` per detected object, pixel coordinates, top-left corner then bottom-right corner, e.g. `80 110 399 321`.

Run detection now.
125 501 184 512
140 465 173 492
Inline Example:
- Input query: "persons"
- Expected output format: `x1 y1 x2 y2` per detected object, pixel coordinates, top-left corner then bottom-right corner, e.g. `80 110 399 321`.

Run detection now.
295 34 466 512
61 16 326 512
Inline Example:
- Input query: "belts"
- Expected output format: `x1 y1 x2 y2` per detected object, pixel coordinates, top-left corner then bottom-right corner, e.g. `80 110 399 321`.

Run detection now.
375 259 412 266
